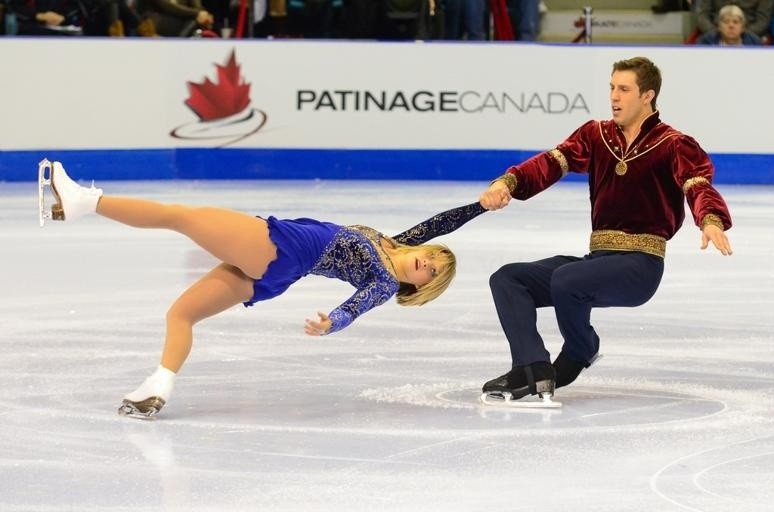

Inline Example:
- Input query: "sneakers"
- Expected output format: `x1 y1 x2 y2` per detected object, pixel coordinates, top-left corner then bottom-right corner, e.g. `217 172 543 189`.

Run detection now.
121 366 176 407
550 337 598 388
52 161 103 223
482 363 556 392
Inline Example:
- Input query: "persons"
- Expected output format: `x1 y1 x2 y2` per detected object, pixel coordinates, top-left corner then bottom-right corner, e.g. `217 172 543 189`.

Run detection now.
479 57 732 399
694 4 763 46
691 0 774 36
49 161 512 412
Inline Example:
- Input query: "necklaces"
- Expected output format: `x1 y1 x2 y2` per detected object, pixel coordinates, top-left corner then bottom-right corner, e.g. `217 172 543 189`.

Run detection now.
614 122 662 176
